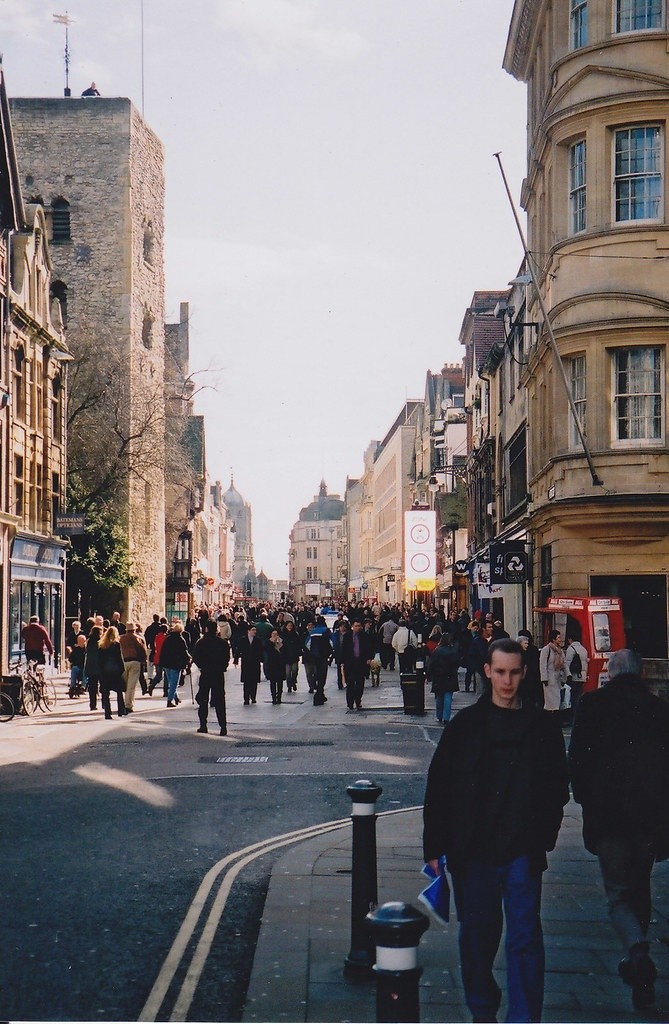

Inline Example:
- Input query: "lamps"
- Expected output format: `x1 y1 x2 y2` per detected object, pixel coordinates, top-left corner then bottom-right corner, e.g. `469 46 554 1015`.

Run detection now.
509 275 533 285
429 463 466 491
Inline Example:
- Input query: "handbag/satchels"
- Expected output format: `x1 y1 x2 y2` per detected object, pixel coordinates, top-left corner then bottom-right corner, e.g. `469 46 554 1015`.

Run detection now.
405 644 416 661
570 645 582 674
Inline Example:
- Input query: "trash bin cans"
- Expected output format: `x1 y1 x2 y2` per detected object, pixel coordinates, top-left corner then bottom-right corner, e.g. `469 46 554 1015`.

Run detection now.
0 675 23 714
400 673 426 716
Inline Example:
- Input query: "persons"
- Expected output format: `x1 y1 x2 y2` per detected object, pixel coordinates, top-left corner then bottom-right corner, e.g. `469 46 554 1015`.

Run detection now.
515 630 588 727
68 614 511 736
82 81 102 97
189 599 496 629
423 639 571 1023
21 616 53 673
568 648 669 1024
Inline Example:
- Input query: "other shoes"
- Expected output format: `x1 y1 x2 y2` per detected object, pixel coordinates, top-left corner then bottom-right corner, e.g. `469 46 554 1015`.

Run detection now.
632 957 656 1007
142 690 149 695
619 957 634 981
149 685 154 696
197 726 207 732
105 715 112 718
220 729 227 736
118 712 128 716
176 699 180 704
244 686 326 705
126 708 133 712
163 692 168 697
91 708 97 710
167 703 176 707
357 703 362 708
349 705 353 709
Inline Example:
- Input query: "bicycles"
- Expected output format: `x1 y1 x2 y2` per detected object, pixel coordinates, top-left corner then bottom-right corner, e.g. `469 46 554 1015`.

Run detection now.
8 659 57 716
0 678 15 722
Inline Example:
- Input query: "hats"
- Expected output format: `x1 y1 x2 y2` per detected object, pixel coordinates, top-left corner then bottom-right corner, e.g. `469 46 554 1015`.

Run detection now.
124 622 139 630
